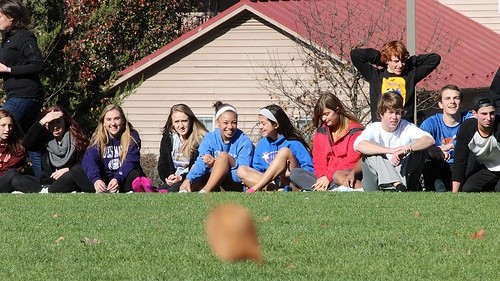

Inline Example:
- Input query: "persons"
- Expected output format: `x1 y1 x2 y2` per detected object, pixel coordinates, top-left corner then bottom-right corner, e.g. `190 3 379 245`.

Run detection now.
332 158 365 193
236 105 314 193
450 95 500 194
352 91 436 194
0 0 47 144
70 103 146 195
0 109 26 193
179 101 256 193
418 85 474 193
350 40 442 123
131 103 210 194
290 92 367 193
22 105 90 195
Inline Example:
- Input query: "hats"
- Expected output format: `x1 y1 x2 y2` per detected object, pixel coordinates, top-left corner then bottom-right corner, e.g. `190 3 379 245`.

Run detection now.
471 95 496 109
